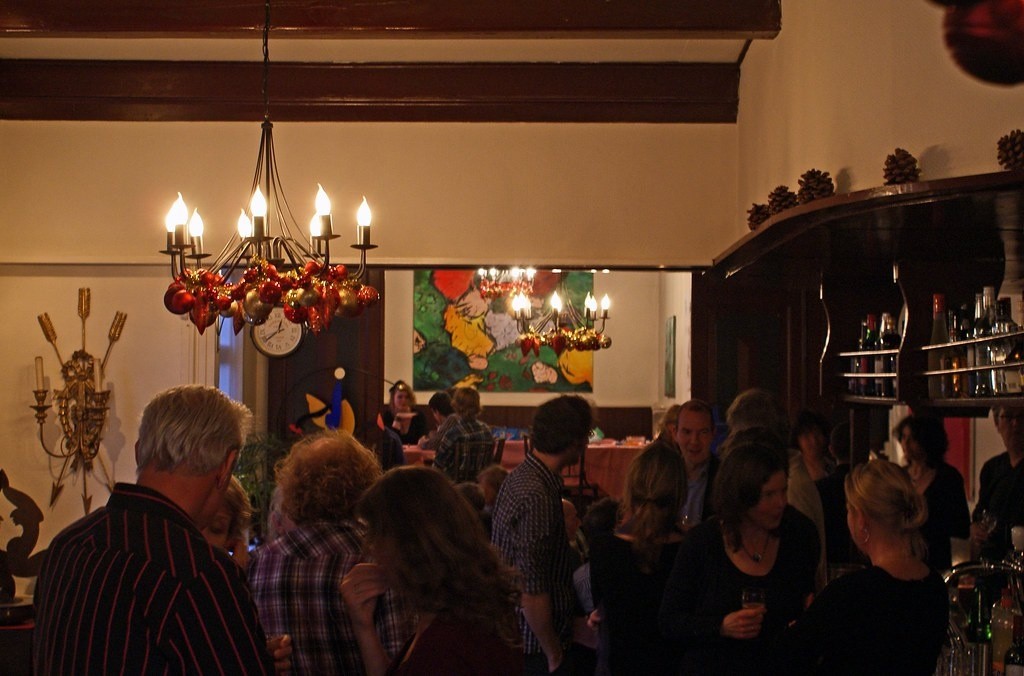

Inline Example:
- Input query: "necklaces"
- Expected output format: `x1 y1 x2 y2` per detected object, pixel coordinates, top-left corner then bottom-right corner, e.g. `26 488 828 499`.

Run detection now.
740 528 768 563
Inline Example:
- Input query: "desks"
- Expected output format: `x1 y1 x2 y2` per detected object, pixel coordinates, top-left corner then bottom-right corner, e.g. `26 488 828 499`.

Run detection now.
569 440 653 525
398 436 529 473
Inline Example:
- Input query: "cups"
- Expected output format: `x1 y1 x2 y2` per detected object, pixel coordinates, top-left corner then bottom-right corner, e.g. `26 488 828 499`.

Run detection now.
677 510 699 534
970 507 997 546
261 633 282 660
739 587 764 612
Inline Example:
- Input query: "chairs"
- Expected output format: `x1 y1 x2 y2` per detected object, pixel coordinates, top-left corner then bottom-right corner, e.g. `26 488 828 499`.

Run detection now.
453 435 507 481
523 432 598 518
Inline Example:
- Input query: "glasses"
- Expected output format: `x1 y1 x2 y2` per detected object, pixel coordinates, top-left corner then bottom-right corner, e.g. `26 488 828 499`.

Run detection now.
996 413 1024 421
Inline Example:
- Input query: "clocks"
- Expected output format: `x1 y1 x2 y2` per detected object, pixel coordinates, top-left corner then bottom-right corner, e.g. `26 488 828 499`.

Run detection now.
249 306 305 357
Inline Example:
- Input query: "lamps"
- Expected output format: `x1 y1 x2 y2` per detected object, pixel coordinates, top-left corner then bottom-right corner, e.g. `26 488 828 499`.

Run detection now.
475 266 538 296
508 271 612 355
150 0 386 335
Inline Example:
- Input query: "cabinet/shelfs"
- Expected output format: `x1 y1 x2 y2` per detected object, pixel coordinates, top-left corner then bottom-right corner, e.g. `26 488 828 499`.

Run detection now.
817 258 1024 410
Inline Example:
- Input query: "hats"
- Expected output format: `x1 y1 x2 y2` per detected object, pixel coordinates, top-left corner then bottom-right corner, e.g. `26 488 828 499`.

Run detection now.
389 380 411 392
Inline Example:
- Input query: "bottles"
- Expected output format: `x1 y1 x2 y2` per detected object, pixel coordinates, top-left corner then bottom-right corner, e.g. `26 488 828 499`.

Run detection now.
969 577 1024 676
849 286 1024 398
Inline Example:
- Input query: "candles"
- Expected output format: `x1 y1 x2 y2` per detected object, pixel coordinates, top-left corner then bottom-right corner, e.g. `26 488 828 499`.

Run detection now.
33 356 45 390
93 358 102 393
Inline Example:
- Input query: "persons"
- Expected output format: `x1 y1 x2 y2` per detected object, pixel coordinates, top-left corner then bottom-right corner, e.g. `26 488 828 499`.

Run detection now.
491 394 600 676
245 424 528 676
972 399 1024 560
589 386 972 676
457 481 486 511
389 379 425 445
383 403 407 470
560 498 583 569
433 387 495 483
32 383 294 676
475 464 509 519
446 388 458 398
417 391 458 450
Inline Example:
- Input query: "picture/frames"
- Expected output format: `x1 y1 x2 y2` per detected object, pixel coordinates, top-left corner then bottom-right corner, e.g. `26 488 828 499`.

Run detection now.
662 315 677 399
